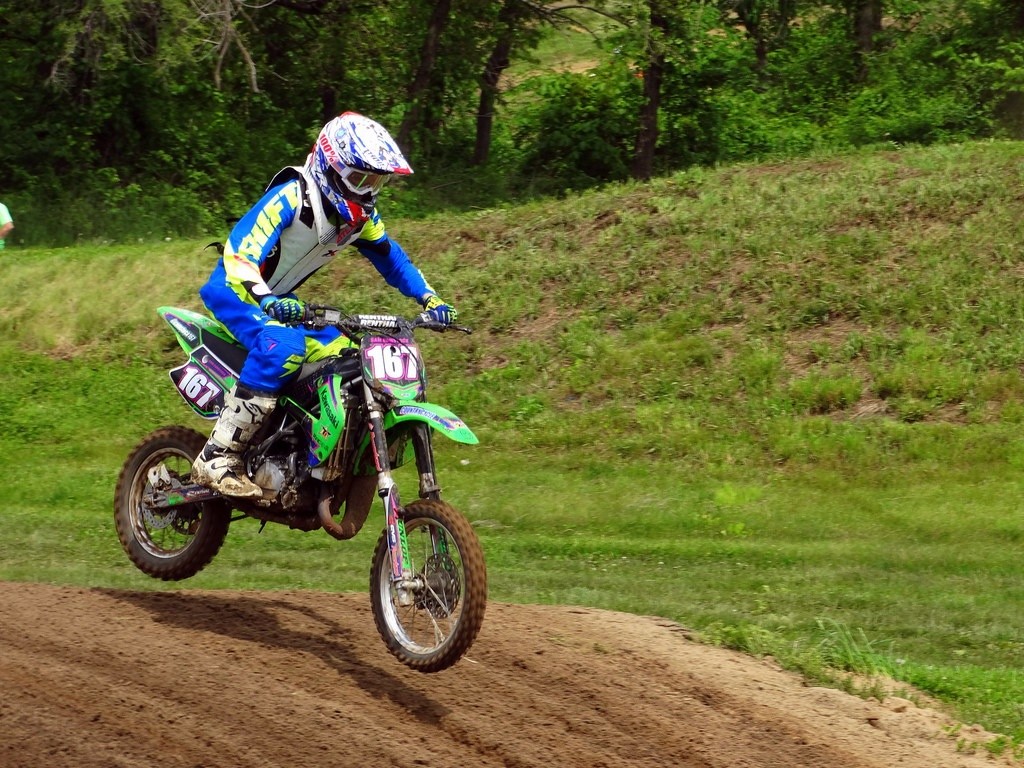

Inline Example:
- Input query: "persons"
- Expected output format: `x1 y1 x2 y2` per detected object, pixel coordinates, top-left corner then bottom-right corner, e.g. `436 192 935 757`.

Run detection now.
189 112 456 498
0 202 13 264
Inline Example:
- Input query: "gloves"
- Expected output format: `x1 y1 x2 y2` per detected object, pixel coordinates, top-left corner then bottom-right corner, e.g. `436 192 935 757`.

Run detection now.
266 298 307 327
424 295 459 326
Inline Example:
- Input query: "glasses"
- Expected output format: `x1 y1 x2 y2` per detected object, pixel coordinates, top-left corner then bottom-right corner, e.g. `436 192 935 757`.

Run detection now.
341 168 391 197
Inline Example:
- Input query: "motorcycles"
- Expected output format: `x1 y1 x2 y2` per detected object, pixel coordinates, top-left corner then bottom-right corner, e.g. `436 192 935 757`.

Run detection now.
109 304 489 674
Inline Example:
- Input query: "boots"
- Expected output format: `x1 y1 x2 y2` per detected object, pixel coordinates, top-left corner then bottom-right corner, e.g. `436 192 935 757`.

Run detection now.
190 383 279 498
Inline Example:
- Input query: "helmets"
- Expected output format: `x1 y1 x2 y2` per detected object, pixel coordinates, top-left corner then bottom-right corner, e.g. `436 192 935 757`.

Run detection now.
308 112 414 223
